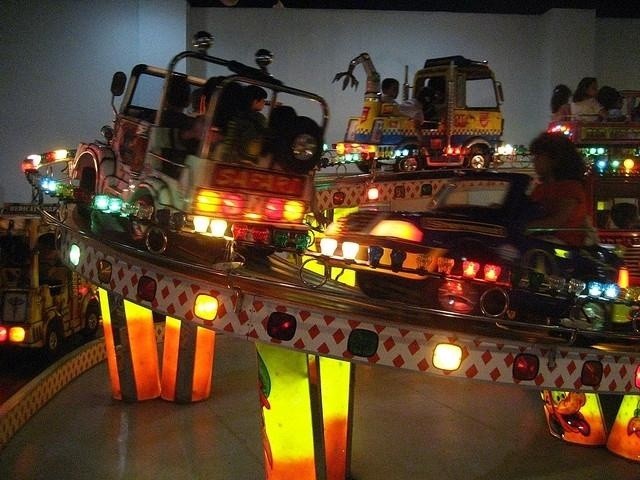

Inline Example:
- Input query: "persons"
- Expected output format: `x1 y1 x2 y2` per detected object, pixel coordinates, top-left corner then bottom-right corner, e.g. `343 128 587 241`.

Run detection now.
24 233 57 295
610 202 639 229
134 76 297 144
382 76 436 127
551 76 632 122
520 131 588 249
